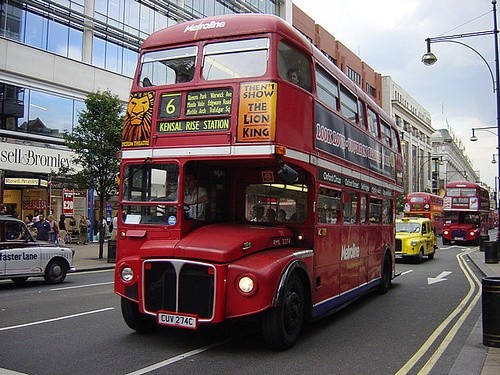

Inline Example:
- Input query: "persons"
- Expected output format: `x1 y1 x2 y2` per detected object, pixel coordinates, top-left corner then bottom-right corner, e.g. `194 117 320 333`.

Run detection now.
287 67 304 88
177 73 187 83
143 78 153 87
59 214 67 243
103 212 118 240
77 216 90 245
0 204 20 239
22 214 59 243
330 209 355 224
251 204 305 222
150 171 219 219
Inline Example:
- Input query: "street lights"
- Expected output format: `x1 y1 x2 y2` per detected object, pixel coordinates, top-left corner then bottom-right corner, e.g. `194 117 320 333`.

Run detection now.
444 163 468 184
416 145 444 192
420 0 500 261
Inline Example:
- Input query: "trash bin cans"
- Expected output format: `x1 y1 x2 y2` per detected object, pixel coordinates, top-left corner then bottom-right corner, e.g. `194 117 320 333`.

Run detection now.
481 276 500 349
107 239 117 263
485 240 498 263
479 235 489 252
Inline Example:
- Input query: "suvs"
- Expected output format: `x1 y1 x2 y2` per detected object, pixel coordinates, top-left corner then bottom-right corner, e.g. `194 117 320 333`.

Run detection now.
0 215 77 285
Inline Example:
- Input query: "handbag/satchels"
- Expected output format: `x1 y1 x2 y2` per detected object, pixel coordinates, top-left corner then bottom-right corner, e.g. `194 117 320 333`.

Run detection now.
54 226 59 234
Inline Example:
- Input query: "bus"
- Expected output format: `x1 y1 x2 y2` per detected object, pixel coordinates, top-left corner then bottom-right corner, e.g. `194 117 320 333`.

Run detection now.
489 207 500 230
440 181 491 246
404 192 443 236
113 12 404 351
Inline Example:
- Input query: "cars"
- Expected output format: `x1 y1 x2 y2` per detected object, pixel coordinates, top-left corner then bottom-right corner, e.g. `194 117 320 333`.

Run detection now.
394 217 438 264
